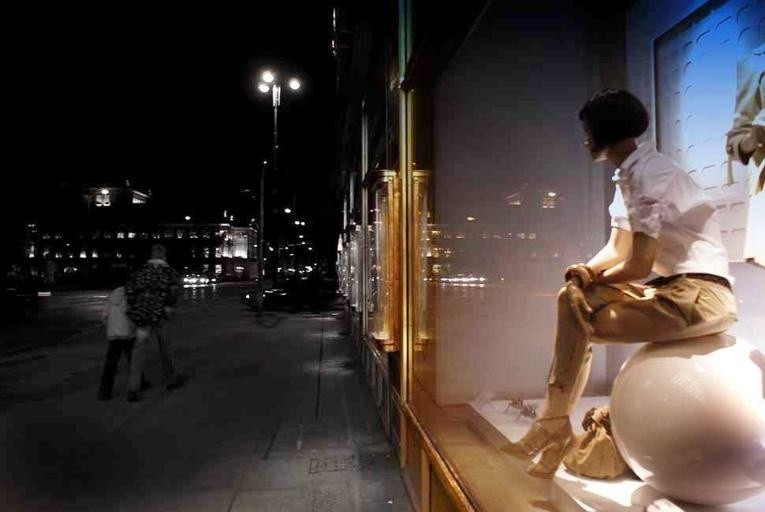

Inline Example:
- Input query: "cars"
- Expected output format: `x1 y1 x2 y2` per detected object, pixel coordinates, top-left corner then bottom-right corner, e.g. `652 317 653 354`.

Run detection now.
241 275 323 312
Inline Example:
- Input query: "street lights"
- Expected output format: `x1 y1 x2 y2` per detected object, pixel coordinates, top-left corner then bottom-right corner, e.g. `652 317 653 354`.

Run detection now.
256 68 302 287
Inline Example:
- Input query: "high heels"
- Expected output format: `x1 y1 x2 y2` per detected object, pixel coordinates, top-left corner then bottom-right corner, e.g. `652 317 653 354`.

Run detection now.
498 416 581 480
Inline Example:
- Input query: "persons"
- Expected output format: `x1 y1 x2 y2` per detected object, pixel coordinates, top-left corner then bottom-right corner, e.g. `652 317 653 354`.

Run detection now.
125 243 184 403
97 270 149 402
726 44 764 270
500 87 741 480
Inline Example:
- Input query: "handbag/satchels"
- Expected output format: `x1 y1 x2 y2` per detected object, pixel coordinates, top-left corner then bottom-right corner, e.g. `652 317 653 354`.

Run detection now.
562 406 629 478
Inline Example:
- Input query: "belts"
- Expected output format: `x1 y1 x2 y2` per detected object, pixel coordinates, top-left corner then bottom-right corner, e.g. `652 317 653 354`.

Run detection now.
661 273 731 288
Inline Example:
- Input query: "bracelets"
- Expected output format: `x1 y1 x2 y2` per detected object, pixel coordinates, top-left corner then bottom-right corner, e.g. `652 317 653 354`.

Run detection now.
562 261 598 291
